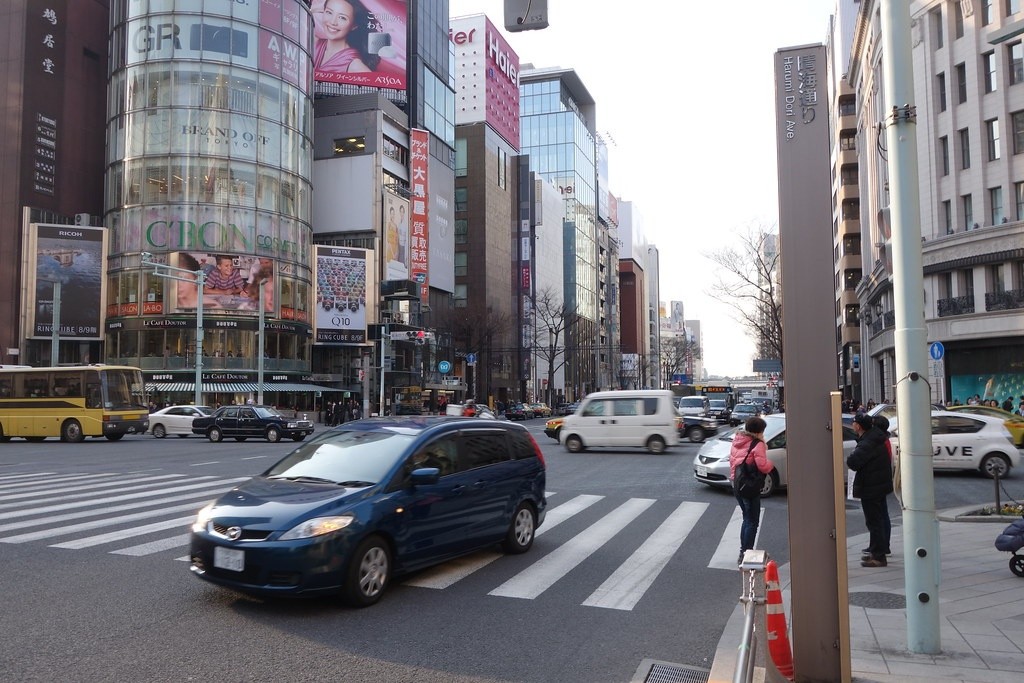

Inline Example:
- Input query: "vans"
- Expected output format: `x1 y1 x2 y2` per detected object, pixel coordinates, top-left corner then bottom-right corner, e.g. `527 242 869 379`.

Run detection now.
560 390 680 454
679 396 710 419
190 414 547 608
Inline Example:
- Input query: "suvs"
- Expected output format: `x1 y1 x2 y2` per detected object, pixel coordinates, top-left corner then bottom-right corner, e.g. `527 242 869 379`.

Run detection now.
709 399 730 424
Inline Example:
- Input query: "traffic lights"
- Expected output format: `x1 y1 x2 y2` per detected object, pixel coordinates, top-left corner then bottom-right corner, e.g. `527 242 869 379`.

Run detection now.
359 370 364 382
405 331 425 343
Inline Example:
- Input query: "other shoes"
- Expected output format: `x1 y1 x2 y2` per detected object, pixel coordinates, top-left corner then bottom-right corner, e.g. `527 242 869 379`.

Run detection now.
862 555 870 561
885 549 892 557
861 557 887 567
862 546 871 553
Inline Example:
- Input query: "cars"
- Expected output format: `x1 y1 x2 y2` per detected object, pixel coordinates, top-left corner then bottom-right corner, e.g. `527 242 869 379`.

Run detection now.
692 402 1024 498
676 416 718 444
504 402 552 420
730 398 779 427
146 405 216 438
192 406 315 443
543 402 686 443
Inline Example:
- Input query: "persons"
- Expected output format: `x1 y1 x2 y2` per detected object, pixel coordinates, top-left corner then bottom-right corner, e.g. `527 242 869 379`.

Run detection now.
849 398 896 413
231 399 238 406
325 399 362 427
313 0 384 75
237 264 274 311
203 255 245 295
178 252 224 308
939 394 1024 418
264 349 281 370
240 258 273 299
496 400 502 415
846 414 893 567
203 349 243 368
729 417 775 565
142 401 162 414
387 205 408 269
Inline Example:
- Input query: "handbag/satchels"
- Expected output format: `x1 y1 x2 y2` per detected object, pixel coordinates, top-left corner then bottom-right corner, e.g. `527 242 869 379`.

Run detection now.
734 438 767 498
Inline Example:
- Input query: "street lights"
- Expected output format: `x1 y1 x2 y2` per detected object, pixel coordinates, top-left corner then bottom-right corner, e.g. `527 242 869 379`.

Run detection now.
258 279 269 405
141 252 207 405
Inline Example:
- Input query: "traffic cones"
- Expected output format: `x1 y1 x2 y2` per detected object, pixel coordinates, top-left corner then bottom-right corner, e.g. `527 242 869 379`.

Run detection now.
766 560 794 681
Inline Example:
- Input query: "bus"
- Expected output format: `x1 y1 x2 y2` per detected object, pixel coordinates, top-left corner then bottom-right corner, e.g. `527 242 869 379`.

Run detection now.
701 386 735 408
0 363 158 443
